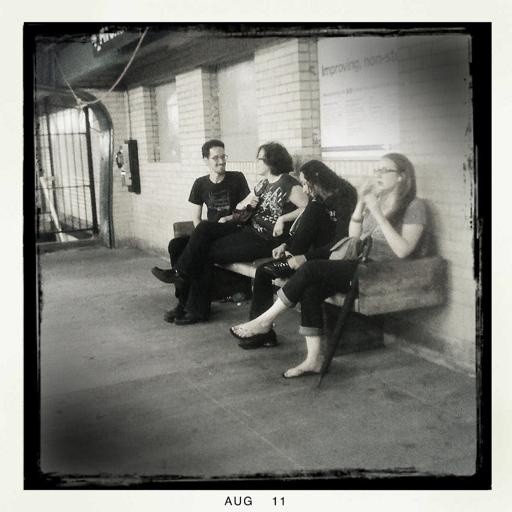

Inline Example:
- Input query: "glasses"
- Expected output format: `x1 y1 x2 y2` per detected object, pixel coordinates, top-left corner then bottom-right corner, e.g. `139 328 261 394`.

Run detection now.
373 167 397 174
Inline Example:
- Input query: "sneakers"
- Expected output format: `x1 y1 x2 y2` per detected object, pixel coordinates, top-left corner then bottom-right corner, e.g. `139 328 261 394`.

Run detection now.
152 266 184 288
262 255 294 278
239 330 276 349
164 305 207 325
223 292 245 303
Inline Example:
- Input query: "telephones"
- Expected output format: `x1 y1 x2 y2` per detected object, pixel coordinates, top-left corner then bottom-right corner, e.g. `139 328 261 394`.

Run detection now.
116 150 122 168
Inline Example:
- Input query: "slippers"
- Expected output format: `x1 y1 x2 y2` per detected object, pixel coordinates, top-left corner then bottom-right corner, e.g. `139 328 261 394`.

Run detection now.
283 367 318 379
229 325 258 339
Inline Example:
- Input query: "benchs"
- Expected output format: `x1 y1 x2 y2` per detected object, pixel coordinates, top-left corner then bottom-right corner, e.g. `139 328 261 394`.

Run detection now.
172 219 449 351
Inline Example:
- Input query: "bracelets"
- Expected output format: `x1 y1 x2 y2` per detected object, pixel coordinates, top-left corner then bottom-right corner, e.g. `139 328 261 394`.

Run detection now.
349 215 363 224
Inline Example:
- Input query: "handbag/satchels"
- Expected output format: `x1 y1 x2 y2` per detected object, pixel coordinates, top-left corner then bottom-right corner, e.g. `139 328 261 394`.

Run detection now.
232 205 253 222
329 236 361 261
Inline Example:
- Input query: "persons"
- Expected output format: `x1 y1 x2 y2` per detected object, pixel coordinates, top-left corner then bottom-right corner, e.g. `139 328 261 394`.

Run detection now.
163 140 251 325
150 141 308 327
228 152 426 377
239 159 360 351
